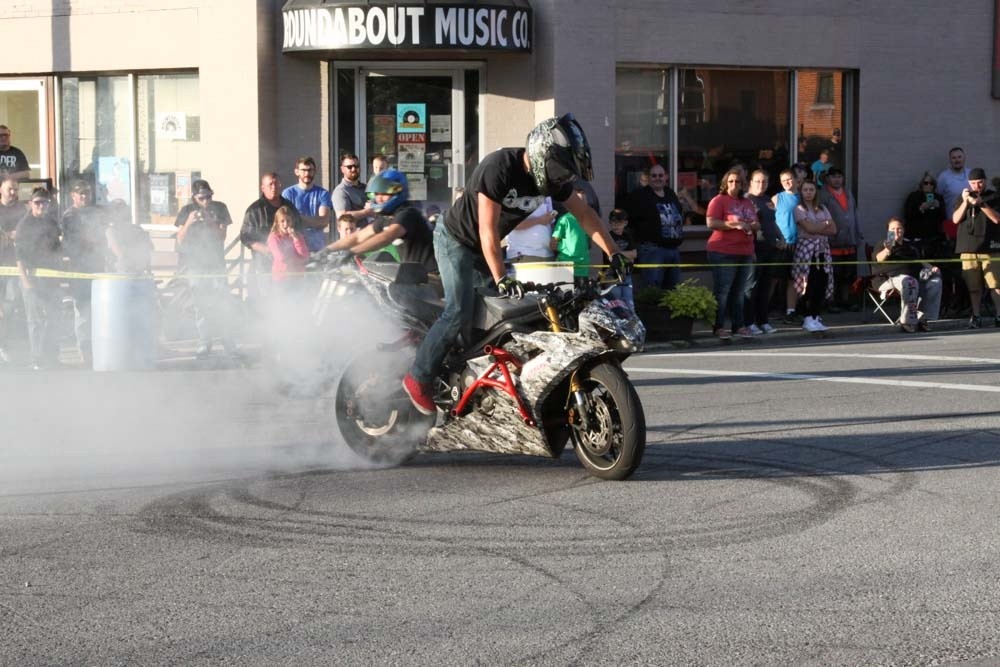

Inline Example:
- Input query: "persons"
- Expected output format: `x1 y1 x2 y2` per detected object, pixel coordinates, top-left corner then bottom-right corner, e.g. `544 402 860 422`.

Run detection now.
706 162 866 339
1 125 151 370
798 127 841 185
871 217 943 333
401 112 634 416
506 182 600 287
175 179 236 359
903 147 1000 329
239 152 433 285
603 165 684 302
678 143 788 225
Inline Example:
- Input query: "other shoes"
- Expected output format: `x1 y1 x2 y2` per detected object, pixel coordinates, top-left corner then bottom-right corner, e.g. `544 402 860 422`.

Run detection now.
715 323 777 338
902 324 915 332
995 316 1000 327
784 311 802 325
828 302 840 313
802 316 829 331
969 315 982 329
918 322 932 331
839 299 859 312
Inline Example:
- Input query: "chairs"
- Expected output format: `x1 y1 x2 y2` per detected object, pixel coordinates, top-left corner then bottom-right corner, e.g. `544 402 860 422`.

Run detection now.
864 240 922 326
943 219 993 318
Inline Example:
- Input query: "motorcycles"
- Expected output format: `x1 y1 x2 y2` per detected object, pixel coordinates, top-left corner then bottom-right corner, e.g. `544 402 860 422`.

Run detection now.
274 252 647 482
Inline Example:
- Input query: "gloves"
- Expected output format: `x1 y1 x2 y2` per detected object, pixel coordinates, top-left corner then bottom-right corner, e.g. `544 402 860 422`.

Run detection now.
496 275 527 299
609 253 633 283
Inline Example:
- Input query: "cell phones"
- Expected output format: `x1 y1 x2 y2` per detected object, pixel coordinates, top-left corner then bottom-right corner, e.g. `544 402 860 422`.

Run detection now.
887 231 894 242
926 194 934 202
550 210 558 216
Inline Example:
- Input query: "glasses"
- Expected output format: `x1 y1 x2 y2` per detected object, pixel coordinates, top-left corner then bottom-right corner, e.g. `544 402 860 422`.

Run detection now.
197 196 212 200
546 159 577 189
342 163 359 169
652 174 663 177
924 182 934 185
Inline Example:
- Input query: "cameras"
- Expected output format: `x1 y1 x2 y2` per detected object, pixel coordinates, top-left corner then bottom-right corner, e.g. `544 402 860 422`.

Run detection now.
969 191 978 199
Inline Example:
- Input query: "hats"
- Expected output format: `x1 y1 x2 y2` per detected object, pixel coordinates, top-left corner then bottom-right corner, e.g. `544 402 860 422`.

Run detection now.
968 168 985 179
31 189 50 202
609 208 628 220
72 180 93 194
191 180 212 195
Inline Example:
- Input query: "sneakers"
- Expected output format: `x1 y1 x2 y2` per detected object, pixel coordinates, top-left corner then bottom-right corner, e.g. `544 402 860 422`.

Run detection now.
402 373 437 416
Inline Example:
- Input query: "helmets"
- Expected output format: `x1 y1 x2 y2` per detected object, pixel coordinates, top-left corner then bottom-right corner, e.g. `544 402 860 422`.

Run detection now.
371 169 408 214
526 113 593 197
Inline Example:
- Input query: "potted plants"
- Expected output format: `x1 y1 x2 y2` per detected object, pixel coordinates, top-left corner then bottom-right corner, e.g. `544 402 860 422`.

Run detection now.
636 276 720 341
